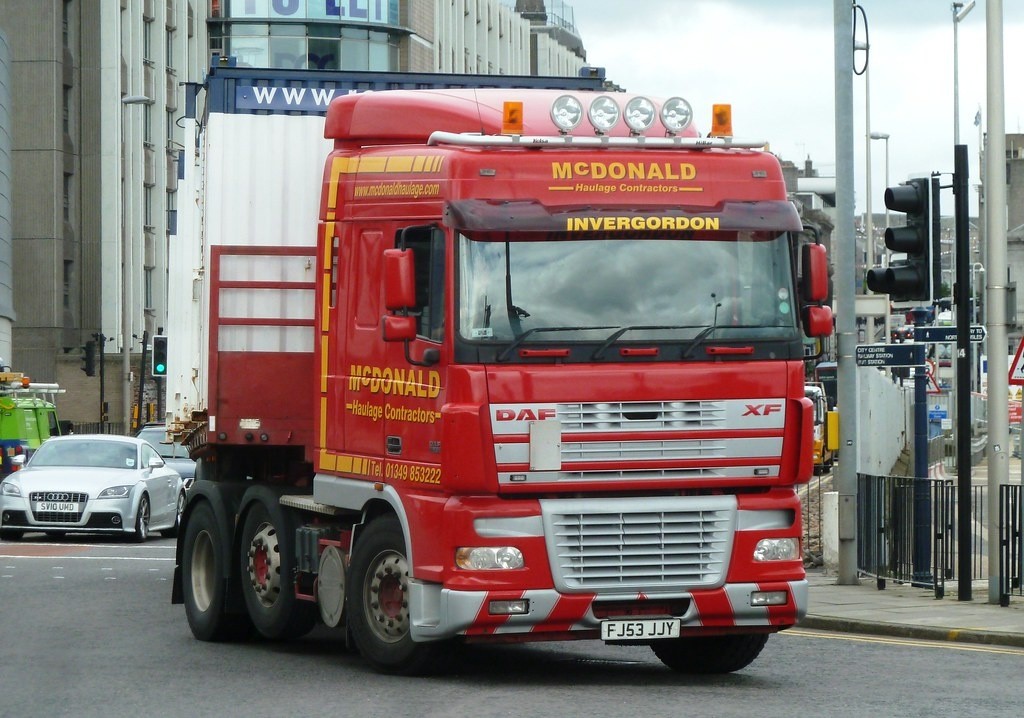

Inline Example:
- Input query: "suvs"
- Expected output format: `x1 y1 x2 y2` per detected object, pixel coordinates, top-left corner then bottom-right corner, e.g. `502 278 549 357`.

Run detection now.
134 421 197 491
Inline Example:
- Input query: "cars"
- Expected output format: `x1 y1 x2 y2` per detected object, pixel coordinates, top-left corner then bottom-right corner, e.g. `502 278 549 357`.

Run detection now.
892 324 914 341
0 433 186 543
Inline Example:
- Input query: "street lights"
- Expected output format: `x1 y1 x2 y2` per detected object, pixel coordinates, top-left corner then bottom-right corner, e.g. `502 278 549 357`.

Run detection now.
972 260 986 434
948 0 980 144
865 132 892 343
852 38 876 344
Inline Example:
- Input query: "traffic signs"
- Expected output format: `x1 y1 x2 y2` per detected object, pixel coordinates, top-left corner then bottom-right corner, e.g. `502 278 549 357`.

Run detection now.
915 327 988 341
854 343 926 367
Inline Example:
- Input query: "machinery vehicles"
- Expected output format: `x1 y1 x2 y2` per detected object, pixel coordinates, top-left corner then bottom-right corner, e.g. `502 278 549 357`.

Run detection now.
0 363 68 488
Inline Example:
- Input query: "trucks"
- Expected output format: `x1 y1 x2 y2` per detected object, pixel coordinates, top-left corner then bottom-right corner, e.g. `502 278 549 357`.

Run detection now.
158 52 835 675
805 381 834 476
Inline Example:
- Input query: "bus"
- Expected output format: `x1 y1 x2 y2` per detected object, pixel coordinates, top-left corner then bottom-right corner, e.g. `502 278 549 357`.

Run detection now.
812 361 838 411
929 312 952 366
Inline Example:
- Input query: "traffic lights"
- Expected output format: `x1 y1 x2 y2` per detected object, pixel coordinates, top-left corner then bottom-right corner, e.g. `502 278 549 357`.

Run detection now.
102 401 109 413
102 415 109 421
152 335 168 377
867 177 931 303
132 402 154 429
79 340 97 377
